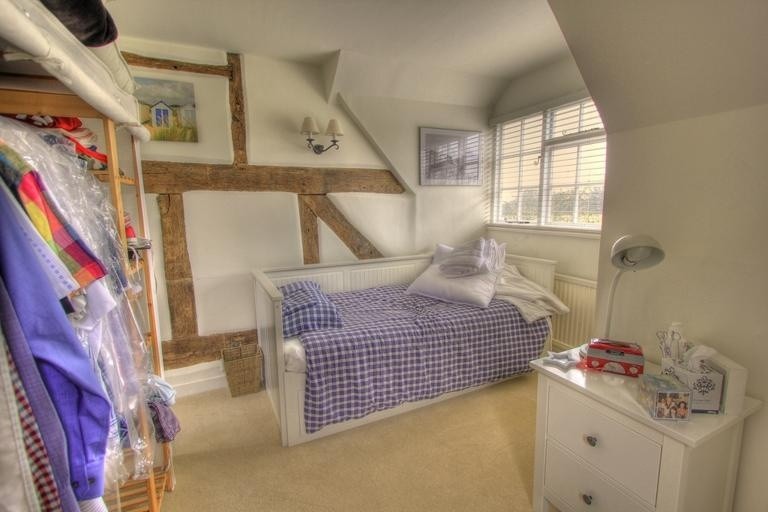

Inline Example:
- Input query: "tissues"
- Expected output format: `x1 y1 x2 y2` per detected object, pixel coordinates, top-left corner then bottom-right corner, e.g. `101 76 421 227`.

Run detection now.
659 344 725 416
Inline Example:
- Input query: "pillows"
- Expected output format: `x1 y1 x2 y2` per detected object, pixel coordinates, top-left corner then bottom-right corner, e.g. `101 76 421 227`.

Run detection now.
402 264 495 309
432 245 453 264
276 281 343 338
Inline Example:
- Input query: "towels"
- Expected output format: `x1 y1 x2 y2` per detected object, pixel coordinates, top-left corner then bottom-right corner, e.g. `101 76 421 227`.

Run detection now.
440 237 506 278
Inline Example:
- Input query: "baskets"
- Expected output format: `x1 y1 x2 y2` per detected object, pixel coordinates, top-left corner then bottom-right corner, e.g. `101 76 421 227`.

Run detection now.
221 343 264 397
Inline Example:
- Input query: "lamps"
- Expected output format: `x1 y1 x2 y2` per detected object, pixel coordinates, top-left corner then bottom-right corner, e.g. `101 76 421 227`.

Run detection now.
298 114 343 157
578 232 667 363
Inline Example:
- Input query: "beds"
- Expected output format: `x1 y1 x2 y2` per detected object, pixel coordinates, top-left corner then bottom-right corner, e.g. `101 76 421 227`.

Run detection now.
251 250 558 451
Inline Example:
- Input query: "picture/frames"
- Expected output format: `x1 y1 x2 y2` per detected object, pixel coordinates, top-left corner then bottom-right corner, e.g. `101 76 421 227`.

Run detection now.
418 125 484 187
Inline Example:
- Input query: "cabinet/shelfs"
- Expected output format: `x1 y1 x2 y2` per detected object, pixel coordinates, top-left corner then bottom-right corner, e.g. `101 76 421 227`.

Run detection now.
528 339 764 511
2 92 175 510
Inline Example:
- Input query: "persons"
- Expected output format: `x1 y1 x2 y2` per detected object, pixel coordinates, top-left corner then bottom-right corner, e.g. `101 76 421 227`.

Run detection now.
656 391 688 421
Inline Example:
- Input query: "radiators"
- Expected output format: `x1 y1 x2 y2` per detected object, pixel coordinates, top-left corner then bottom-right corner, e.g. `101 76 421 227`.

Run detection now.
550 270 596 354
